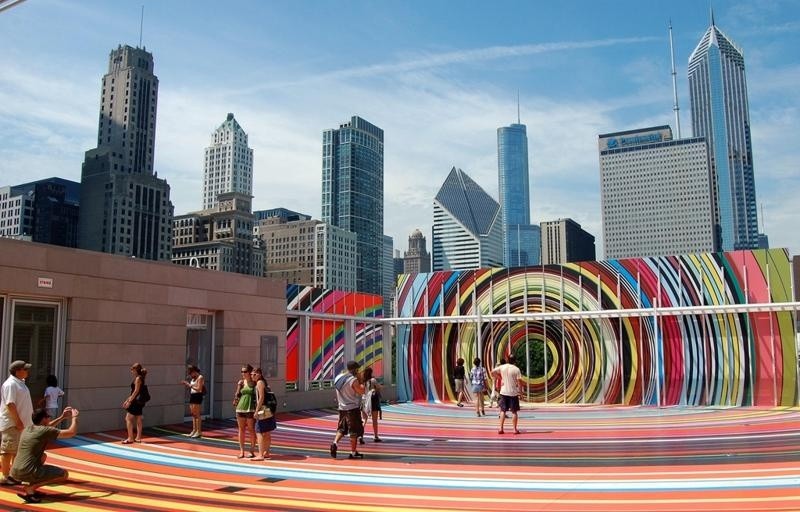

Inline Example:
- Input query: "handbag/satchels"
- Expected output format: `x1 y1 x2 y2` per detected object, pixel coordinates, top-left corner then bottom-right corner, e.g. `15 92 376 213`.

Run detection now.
202 384 208 396
140 383 152 403
254 405 273 421
483 386 488 395
233 397 239 406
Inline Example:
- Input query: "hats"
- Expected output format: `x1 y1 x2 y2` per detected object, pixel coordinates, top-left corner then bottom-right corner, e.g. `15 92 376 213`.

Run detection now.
347 360 360 368
9 359 32 373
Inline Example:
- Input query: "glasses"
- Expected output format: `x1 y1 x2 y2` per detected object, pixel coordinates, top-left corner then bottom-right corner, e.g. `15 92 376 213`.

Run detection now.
241 369 248 373
250 373 260 376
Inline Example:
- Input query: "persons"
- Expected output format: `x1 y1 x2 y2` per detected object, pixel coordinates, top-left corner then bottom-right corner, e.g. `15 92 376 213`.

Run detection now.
233 365 256 458
181 365 205 438
10 407 79 503
38 375 64 419
455 357 523 433
250 367 276 461
332 360 382 458
0 361 34 486
122 363 147 444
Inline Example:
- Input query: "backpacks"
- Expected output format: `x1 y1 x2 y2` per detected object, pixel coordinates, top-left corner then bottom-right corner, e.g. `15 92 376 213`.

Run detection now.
264 386 277 412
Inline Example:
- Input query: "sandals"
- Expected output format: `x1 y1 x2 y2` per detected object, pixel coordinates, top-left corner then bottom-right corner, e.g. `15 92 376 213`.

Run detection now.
192 432 202 438
188 431 196 438
457 402 523 434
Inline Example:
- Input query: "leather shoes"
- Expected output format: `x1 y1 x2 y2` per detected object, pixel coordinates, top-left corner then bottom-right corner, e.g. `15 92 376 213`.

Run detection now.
246 454 254 458
1 480 15 486
264 454 270 457
250 457 264 461
239 453 244 458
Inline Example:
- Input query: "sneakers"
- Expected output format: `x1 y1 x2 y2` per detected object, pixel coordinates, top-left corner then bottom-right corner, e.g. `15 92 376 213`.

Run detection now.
330 442 338 459
374 436 381 442
349 450 363 459
358 435 365 445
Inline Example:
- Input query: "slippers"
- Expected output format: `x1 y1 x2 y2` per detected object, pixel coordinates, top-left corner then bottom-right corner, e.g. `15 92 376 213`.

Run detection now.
17 490 43 503
122 439 132 444
134 438 141 443
7 476 22 484
